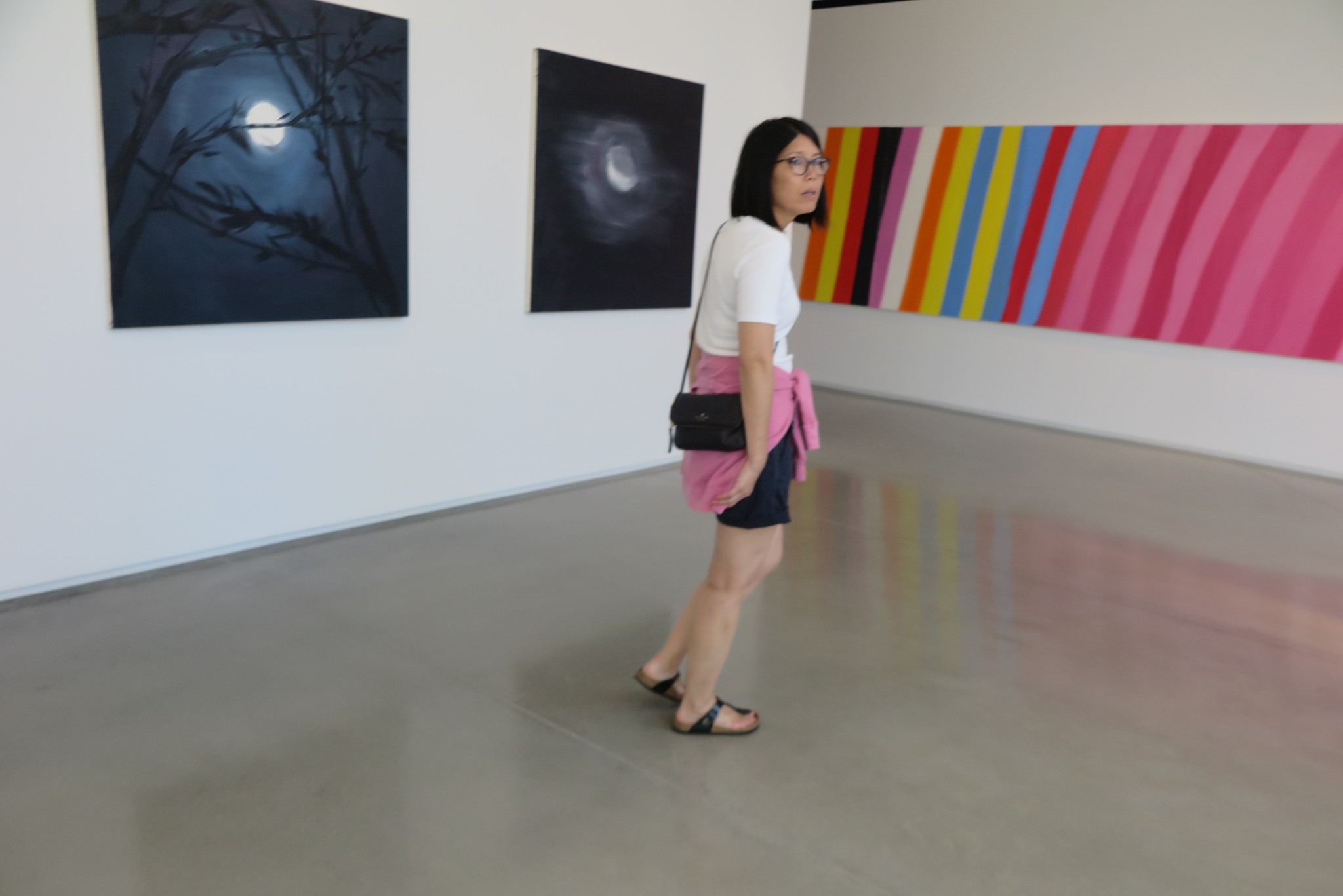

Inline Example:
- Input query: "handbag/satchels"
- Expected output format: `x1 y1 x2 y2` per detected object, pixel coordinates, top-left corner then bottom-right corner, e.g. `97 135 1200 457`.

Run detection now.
667 392 746 453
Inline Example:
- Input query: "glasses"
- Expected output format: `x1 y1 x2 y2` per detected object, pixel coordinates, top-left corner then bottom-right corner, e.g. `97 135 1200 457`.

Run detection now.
775 156 831 177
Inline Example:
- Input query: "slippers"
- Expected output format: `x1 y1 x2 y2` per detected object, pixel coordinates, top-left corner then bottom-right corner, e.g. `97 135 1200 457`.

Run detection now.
676 695 759 736
634 667 686 705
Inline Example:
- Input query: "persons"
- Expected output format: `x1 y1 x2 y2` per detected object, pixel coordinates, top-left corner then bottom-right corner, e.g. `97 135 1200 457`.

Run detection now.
633 117 828 737
